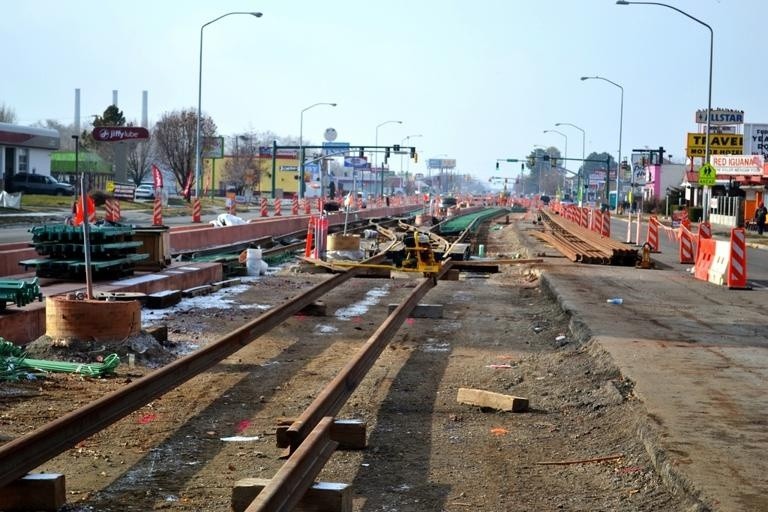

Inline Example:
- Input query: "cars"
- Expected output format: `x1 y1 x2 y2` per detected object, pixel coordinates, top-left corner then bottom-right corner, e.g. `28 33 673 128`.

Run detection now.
540 195 549 203
559 199 574 206
135 184 154 199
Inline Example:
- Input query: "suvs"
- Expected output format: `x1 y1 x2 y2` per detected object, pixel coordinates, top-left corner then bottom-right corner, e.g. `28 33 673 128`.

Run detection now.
11 172 74 196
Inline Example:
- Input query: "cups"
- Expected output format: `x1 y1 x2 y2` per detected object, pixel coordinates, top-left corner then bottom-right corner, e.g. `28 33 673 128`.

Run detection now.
478 244 484 258
130 354 136 369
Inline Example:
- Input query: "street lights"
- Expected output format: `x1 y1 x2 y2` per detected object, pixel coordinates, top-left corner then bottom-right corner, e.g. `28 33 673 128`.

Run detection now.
70 133 79 200
399 135 422 145
234 133 248 164
193 10 263 201
580 76 620 218
616 0 713 225
296 102 338 203
554 122 585 190
532 143 551 174
374 120 402 197
543 129 565 188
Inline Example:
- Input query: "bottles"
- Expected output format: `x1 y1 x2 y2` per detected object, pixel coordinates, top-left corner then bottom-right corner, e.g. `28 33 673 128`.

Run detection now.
606 298 625 304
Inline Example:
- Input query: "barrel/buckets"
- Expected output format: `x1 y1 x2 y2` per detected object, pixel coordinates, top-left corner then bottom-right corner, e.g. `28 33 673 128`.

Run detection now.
239 248 269 276
362 229 378 239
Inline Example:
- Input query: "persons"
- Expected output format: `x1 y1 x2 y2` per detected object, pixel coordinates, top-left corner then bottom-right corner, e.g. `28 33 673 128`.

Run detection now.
752 200 767 236
